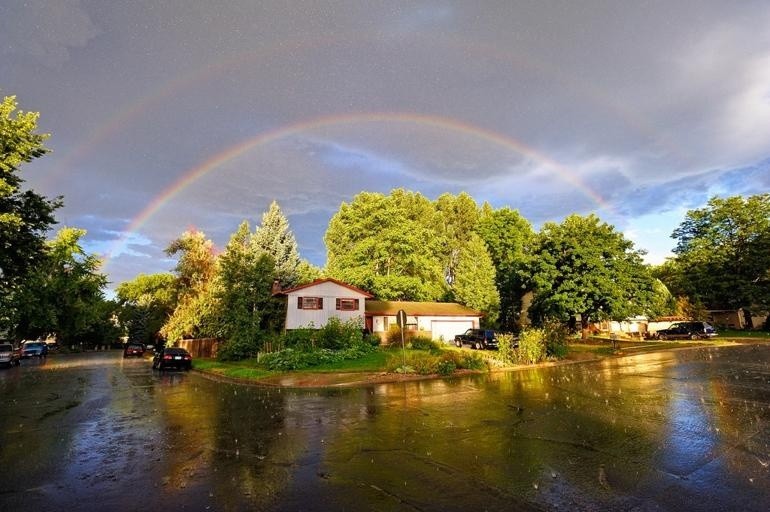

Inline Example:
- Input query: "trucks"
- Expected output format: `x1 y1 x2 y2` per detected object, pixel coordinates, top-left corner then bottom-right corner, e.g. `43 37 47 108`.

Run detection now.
644 320 719 338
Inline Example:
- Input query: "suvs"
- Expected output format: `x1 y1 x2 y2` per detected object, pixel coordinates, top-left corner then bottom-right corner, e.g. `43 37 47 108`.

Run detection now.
655 321 707 341
454 328 516 350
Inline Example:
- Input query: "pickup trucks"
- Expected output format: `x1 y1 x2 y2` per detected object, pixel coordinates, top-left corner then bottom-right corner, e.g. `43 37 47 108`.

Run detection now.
0 343 21 367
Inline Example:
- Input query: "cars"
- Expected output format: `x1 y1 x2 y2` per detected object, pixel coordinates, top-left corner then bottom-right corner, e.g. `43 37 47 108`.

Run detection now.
152 347 192 371
147 344 155 350
47 342 60 351
124 343 143 358
143 343 146 353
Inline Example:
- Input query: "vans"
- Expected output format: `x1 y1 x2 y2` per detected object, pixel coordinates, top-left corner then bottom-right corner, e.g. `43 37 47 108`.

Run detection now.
21 341 47 359
